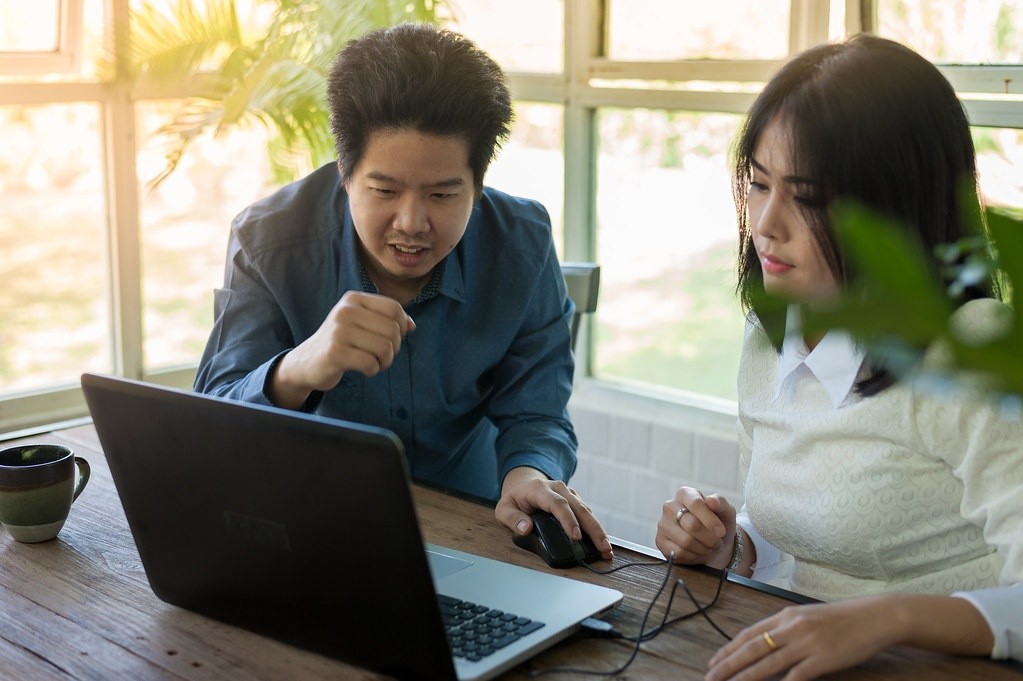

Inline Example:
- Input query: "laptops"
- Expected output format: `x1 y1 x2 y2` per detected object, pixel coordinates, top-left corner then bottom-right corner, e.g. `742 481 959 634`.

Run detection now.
79 372 626 680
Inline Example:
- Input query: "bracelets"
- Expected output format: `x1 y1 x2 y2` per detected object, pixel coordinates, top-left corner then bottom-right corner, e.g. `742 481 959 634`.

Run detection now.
725 528 744 571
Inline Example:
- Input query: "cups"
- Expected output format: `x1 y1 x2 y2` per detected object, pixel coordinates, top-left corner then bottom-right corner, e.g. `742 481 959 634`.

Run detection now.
0 444 92 544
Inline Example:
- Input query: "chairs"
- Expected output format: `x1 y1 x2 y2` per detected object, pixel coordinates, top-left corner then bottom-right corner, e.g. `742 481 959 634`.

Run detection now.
556 261 600 348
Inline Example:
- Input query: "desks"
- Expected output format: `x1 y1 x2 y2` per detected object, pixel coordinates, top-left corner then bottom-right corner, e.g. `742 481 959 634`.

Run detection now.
0 420 1023 681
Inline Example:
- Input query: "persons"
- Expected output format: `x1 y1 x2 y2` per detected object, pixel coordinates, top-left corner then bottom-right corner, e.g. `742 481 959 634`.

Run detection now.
656 33 1023 681
194 21 614 559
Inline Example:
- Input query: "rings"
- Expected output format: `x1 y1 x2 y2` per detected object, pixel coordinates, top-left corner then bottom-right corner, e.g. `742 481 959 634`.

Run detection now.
763 630 777 649
676 507 687 522
670 551 674 556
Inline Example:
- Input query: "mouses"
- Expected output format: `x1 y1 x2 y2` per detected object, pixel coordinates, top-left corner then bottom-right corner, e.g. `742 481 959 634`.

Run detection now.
512 509 605 569
